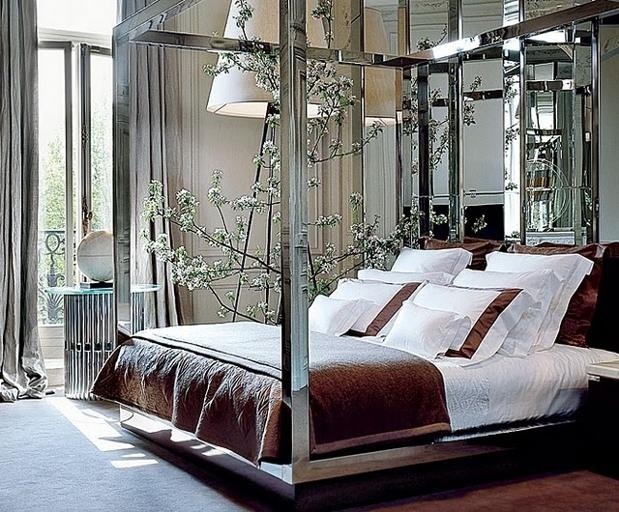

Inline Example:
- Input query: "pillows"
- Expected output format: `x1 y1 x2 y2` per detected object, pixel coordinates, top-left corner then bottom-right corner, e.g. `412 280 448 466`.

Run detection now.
307 238 595 363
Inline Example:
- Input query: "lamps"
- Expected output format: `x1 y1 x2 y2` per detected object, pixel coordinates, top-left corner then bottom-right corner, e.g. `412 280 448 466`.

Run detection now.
77 230 115 288
362 6 402 128
208 0 343 322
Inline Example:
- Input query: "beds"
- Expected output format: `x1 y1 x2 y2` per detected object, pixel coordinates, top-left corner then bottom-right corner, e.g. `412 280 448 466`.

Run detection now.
90 238 619 499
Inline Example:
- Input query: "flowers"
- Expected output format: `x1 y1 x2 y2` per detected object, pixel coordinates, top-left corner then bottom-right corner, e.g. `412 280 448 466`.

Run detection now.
403 25 489 242
140 0 424 324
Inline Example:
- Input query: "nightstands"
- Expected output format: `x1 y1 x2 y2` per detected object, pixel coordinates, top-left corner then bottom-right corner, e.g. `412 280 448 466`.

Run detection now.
584 363 619 480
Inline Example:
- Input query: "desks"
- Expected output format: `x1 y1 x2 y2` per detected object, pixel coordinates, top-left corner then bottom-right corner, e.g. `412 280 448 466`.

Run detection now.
42 284 158 400
